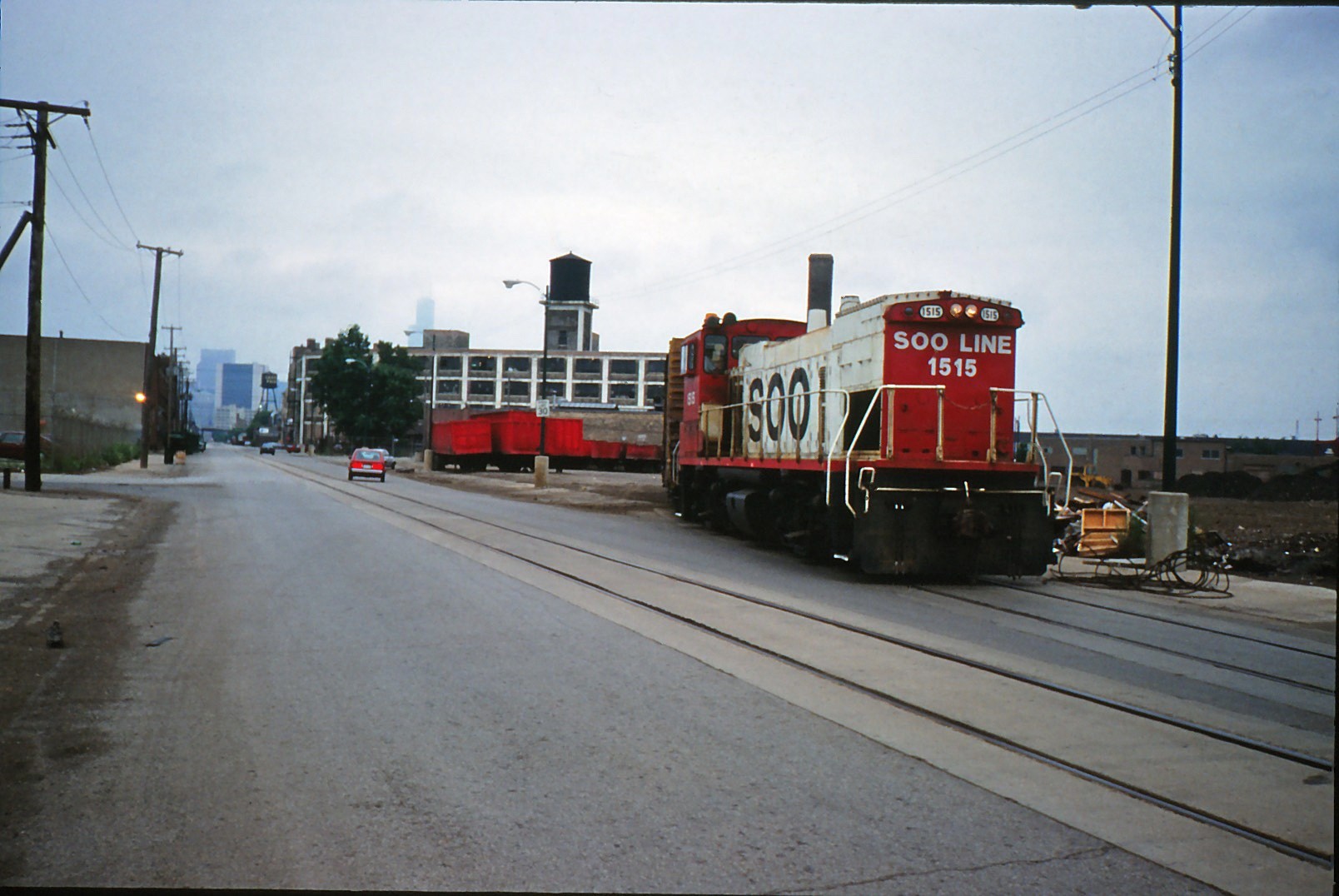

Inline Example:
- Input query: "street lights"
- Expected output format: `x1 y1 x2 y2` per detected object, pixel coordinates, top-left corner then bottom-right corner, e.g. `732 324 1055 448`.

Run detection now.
503 280 549 456
1075 3 1183 488
405 330 435 450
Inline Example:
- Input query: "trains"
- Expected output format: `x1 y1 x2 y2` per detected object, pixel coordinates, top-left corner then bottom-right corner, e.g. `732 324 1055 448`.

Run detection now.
663 254 1075 580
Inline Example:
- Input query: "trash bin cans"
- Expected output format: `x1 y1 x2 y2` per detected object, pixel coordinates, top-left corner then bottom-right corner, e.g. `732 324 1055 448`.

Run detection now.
165 433 184 461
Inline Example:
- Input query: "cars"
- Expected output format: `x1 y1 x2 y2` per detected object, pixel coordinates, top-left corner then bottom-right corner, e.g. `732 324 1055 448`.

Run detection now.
260 444 275 455
348 447 385 482
0 431 54 459
287 444 307 453
377 449 396 470
